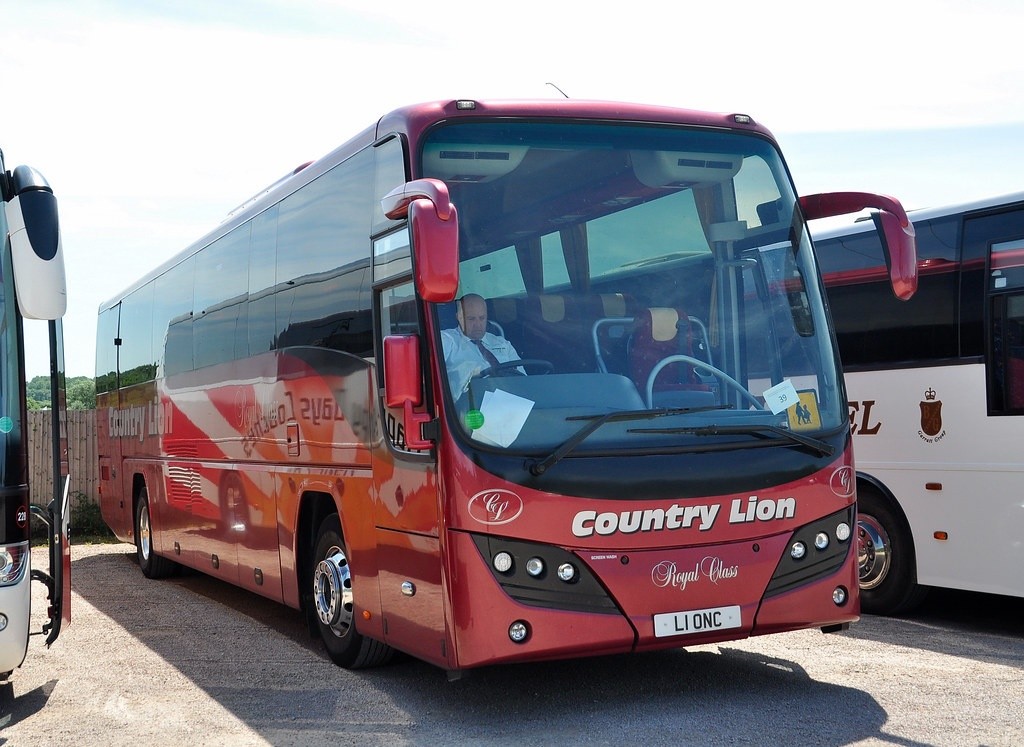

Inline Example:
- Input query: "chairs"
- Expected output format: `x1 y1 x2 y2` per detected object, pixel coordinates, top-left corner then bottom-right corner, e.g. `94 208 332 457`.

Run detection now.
481 289 723 407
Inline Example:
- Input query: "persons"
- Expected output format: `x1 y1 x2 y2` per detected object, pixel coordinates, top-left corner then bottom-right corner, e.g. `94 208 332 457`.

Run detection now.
441 294 527 404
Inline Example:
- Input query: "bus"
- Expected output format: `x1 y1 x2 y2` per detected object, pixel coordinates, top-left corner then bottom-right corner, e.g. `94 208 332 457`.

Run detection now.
0 148 72 685
96 98 920 673
499 191 1024 616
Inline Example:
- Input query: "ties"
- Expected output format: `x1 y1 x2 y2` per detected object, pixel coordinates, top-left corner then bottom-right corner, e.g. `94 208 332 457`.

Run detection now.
469 339 500 369
